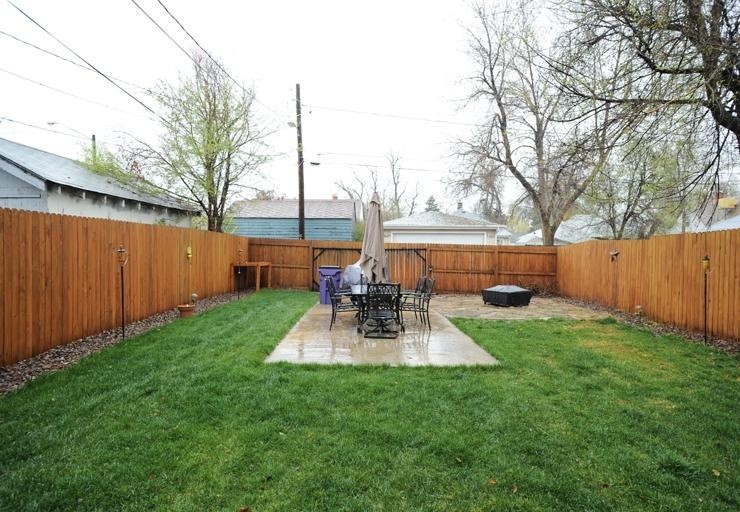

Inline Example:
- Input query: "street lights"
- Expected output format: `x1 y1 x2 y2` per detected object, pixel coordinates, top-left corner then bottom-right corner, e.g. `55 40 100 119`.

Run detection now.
286 121 305 239
46 120 98 163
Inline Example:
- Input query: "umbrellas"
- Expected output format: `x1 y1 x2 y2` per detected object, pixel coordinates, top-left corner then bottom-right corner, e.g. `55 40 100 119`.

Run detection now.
357 190 387 316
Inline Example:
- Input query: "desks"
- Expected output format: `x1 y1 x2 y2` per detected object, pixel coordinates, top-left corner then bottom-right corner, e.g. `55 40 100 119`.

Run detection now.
229 261 273 294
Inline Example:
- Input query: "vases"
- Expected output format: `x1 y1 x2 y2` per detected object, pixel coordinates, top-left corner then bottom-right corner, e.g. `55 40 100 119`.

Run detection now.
177 304 196 319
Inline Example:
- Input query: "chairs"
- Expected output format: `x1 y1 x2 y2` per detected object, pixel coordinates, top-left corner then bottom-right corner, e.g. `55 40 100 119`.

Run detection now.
325 275 436 340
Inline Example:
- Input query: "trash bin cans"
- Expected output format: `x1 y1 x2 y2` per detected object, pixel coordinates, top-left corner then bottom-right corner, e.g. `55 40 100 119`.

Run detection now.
318 268 342 304
